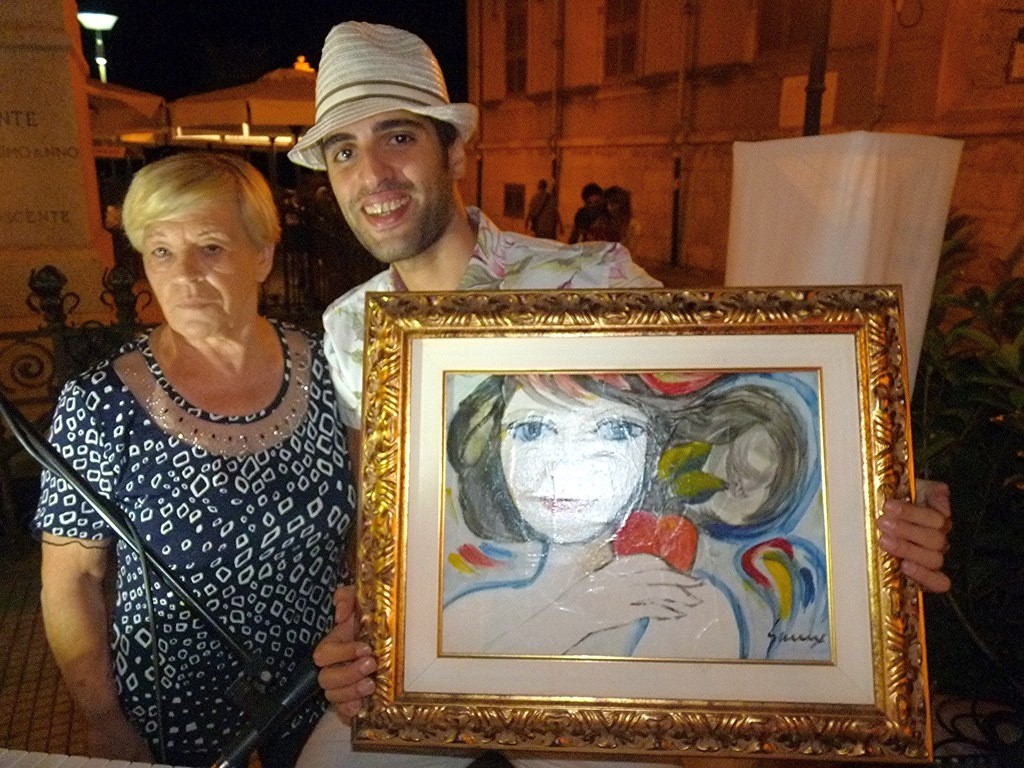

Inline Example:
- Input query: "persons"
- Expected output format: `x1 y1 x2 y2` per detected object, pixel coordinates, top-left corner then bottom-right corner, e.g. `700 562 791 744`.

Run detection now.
34 152 358 768
281 20 950 768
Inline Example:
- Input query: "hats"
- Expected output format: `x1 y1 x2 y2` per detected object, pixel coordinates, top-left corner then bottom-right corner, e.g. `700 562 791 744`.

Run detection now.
286 20 480 172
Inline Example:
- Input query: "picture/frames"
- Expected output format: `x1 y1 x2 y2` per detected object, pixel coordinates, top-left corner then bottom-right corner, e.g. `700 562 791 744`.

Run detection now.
351 284 933 764
